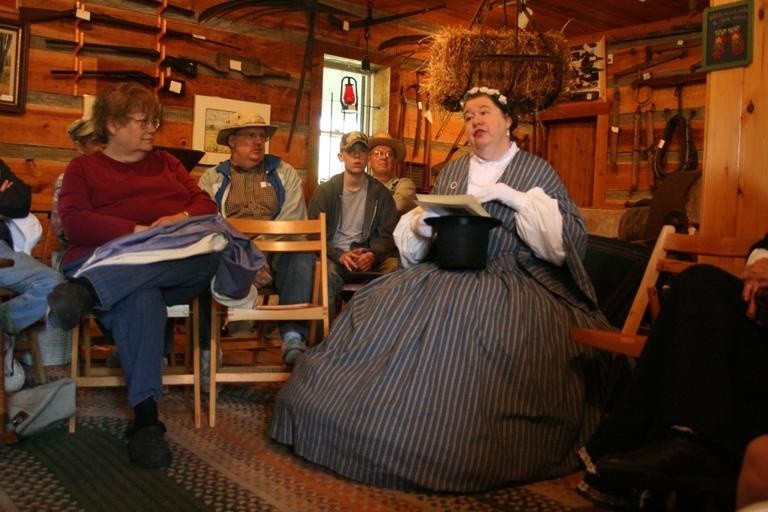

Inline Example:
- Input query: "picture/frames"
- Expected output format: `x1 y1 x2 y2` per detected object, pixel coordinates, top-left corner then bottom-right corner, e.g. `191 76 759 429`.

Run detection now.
192 93 271 167
565 34 607 101
697 1 755 73
1 16 31 116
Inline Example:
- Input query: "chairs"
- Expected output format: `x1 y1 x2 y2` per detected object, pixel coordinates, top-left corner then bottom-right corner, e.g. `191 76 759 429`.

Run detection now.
568 225 761 401
3 285 46 387
68 296 201 432
339 268 386 294
209 212 329 426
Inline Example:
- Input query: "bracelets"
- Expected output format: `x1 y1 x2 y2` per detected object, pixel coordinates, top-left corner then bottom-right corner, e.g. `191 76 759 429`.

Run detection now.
184 211 191 217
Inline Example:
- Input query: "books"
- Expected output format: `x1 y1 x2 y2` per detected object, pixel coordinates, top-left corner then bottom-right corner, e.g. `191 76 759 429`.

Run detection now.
412 192 490 219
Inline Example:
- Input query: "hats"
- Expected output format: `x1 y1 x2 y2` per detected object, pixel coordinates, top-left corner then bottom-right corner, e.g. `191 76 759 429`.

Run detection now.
422 214 503 272
368 131 407 164
339 131 368 152
65 118 97 140
215 111 278 145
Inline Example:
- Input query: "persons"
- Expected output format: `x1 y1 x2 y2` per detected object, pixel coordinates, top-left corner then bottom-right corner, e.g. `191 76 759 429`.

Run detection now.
734 435 768 512
196 111 315 393
48 80 221 474
307 131 402 344
1 159 66 394
574 234 768 512
364 131 418 215
269 86 636 493
50 117 172 392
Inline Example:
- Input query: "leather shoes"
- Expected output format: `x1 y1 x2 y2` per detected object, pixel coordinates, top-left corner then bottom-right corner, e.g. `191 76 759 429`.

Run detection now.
591 434 747 500
125 416 171 472
45 280 97 333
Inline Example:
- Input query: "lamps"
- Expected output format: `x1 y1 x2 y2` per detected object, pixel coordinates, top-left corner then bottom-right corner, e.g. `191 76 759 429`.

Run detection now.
339 77 357 112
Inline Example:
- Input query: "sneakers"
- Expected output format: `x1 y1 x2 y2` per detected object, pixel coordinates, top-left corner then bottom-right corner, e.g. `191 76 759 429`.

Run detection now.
279 337 307 368
201 354 226 395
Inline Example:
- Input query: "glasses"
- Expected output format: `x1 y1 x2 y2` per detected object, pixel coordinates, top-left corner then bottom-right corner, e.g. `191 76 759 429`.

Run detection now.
233 132 266 140
126 116 161 129
370 150 395 159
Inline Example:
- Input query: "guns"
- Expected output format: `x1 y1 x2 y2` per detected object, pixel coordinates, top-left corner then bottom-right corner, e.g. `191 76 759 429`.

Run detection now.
127 0 194 17
568 42 604 100
18 6 241 52
51 69 186 97
44 38 197 77
